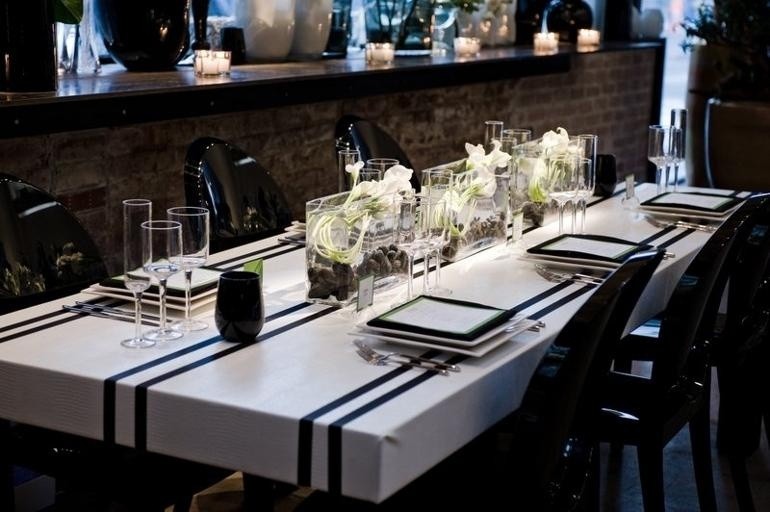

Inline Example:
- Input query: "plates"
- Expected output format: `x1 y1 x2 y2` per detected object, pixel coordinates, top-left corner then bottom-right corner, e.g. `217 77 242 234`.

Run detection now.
79 264 234 313
346 295 540 358
281 219 306 232
517 233 661 273
633 191 748 223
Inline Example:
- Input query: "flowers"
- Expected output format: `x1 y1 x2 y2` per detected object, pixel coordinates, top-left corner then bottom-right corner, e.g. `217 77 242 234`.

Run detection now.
335 157 415 240
439 137 499 228
524 128 586 199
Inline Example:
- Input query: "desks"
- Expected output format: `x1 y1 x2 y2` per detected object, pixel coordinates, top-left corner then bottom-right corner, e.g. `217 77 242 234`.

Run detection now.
0 184 768 505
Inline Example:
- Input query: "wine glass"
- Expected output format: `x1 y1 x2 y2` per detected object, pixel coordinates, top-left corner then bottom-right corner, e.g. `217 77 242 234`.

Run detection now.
645 107 687 196
336 144 456 302
140 220 185 343
477 119 597 244
165 206 211 333
121 196 157 350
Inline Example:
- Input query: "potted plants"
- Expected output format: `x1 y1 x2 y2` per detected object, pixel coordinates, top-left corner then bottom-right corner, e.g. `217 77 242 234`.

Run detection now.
682 0 770 192
363 0 485 56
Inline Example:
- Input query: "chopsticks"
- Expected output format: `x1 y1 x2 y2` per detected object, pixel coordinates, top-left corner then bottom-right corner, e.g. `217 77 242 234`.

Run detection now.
277 237 306 246
62 300 173 328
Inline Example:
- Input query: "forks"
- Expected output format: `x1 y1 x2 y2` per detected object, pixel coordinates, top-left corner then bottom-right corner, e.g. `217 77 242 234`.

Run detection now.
351 338 462 378
643 216 719 234
531 262 604 287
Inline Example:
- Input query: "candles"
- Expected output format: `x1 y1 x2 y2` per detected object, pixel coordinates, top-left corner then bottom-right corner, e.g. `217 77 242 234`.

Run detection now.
365 41 393 65
195 50 231 76
535 31 558 50
578 29 600 46
452 36 481 57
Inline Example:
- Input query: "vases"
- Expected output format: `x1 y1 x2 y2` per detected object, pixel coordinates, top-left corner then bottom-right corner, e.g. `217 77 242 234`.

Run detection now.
512 146 597 228
422 159 521 257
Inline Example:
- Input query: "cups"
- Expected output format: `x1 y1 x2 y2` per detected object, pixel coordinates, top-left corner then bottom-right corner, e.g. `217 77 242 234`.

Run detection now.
192 48 233 76
91 0 191 73
234 1 335 64
576 28 602 53
454 36 481 57
593 153 618 198
532 32 560 55
364 41 395 65
215 272 266 342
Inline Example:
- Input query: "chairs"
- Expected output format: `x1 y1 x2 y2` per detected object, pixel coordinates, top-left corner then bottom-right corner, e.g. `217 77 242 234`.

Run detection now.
602 193 769 512
333 118 422 193
180 136 313 509
421 244 666 510
10 199 117 512
